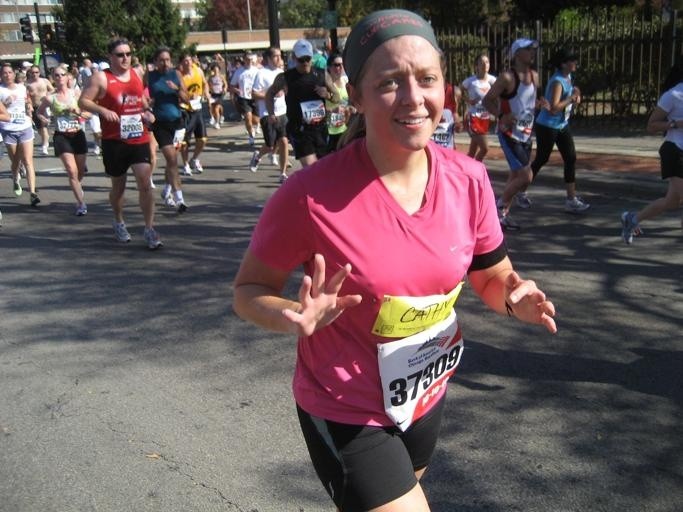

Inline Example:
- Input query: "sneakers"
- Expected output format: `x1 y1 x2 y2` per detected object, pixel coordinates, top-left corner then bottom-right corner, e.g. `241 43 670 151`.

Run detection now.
143 225 164 250
492 190 534 232
160 190 188 212
209 116 224 130
565 197 590 215
249 151 262 172
76 181 87 215
30 194 40 206
267 152 294 168
247 124 262 146
183 164 193 176
189 157 204 174
13 165 26 196
110 220 132 243
41 142 105 175
277 174 289 184
619 210 641 244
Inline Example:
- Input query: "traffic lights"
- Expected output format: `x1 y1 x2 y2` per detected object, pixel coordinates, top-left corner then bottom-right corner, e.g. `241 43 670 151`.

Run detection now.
53 21 67 43
19 13 33 44
40 22 55 50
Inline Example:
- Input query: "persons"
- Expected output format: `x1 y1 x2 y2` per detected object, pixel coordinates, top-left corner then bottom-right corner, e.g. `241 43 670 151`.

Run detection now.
621 64 683 244
459 53 498 162
482 36 549 231
429 48 463 150
231 10 557 512
514 49 590 213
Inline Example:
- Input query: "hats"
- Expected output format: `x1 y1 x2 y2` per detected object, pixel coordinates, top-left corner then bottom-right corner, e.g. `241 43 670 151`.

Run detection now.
293 38 315 61
78 61 110 73
22 61 34 68
512 38 541 54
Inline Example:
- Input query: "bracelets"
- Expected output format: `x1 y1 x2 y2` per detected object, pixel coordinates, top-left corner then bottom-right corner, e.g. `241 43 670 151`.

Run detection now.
505 300 514 319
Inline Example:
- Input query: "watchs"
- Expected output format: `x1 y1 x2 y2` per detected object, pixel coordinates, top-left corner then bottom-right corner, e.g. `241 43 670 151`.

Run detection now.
670 121 678 130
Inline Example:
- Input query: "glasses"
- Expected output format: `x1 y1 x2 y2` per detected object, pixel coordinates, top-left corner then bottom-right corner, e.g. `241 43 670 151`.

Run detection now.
112 52 132 57
